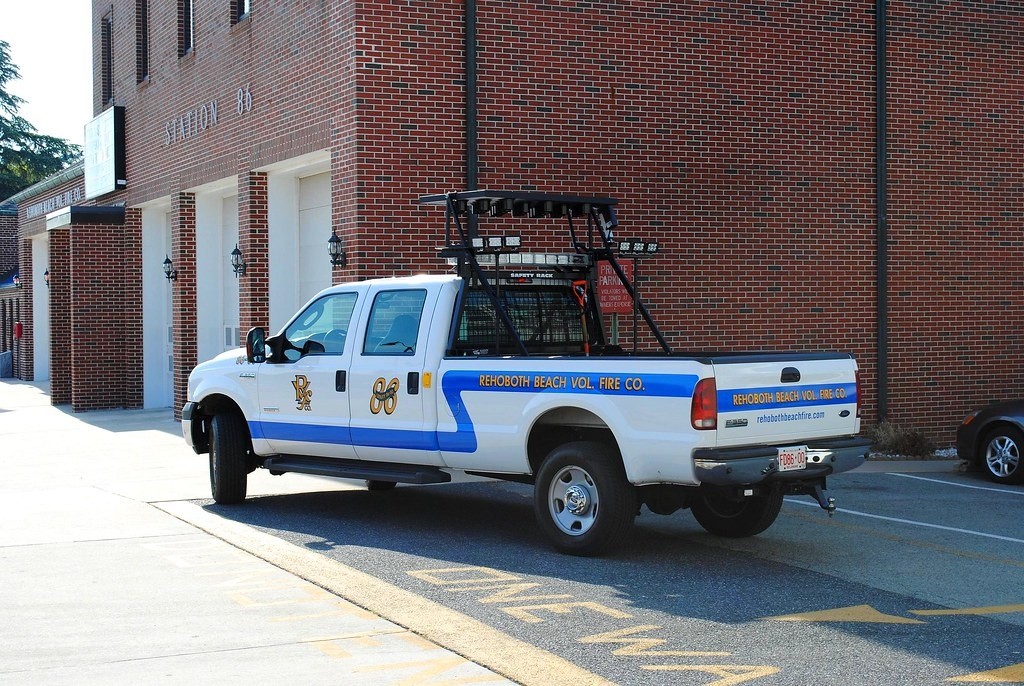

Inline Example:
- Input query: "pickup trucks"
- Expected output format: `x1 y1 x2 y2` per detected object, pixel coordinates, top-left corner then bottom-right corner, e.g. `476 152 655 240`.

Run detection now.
180 274 878 557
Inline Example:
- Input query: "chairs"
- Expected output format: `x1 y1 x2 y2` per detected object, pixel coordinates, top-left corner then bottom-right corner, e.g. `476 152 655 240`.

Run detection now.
373 314 418 354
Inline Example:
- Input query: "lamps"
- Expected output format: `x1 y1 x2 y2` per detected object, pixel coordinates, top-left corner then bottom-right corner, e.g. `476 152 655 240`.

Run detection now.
13 274 22 289
328 229 346 268
162 254 177 283
44 268 49 289
230 243 246 278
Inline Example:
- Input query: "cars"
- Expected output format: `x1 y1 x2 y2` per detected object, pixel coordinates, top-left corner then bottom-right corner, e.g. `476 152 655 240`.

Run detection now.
955 400 1024 486
